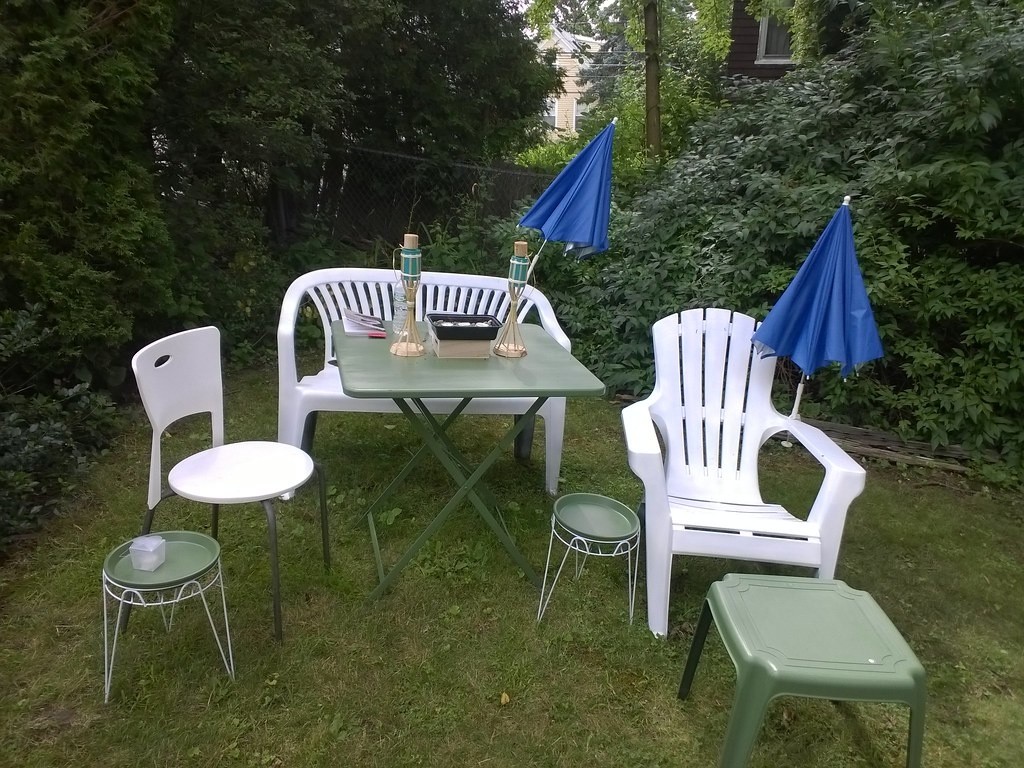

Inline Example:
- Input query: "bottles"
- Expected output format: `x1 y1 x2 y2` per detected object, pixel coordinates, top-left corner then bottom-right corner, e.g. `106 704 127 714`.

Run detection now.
392 279 408 336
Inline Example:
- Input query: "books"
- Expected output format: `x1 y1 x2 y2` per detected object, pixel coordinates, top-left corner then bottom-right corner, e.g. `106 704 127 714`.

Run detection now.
342 308 386 338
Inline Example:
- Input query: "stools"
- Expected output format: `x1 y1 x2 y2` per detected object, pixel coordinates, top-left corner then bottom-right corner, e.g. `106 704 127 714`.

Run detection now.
676 572 928 767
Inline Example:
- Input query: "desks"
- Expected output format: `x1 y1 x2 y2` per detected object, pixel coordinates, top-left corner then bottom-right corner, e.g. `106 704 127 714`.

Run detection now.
333 315 608 605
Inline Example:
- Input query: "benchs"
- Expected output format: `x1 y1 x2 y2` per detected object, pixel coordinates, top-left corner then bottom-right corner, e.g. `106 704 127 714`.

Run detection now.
273 267 572 502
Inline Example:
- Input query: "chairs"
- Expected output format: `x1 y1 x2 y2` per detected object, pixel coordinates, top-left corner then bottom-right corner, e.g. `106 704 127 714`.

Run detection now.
623 308 867 639
121 324 329 645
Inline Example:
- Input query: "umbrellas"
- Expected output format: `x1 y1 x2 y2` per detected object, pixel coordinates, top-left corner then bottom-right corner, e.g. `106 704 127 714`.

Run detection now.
750 196 883 443
516 117 618 283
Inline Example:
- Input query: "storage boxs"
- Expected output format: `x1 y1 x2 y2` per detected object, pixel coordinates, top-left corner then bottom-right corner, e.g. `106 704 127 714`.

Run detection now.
427 313 503 340
129 535 165 571
